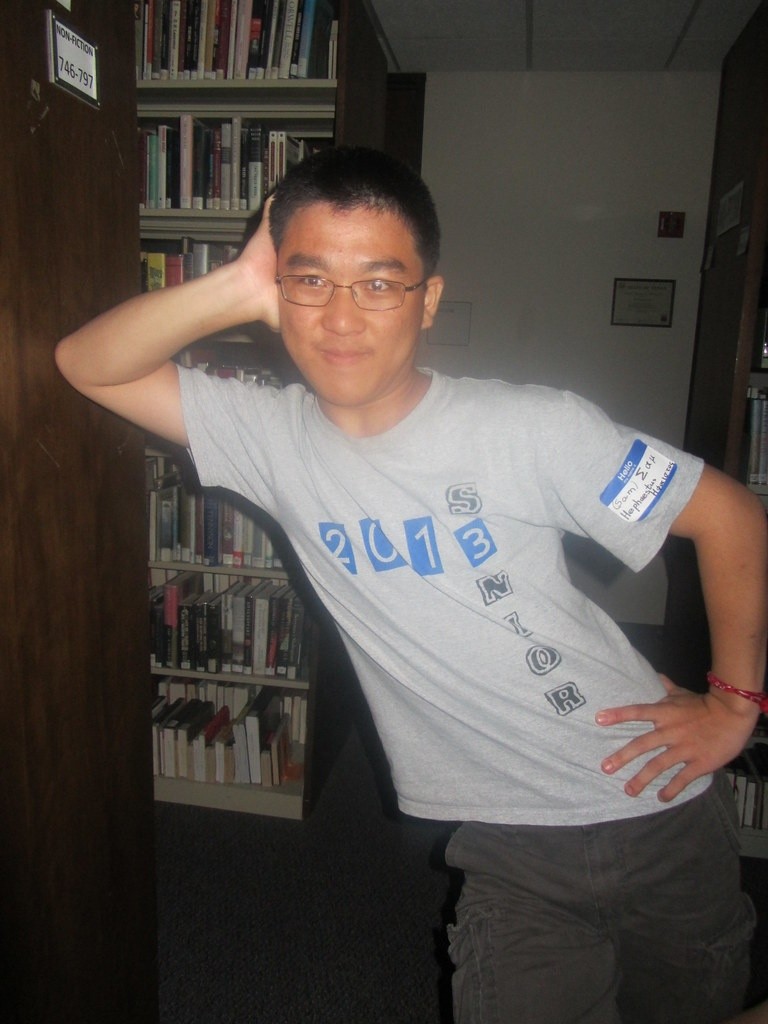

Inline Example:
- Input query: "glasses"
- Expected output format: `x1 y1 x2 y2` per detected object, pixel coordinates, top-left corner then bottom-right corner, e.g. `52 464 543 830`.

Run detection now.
274 273 430 311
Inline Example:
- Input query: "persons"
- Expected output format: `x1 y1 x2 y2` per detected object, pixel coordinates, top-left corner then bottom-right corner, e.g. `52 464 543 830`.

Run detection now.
50 143 768 1023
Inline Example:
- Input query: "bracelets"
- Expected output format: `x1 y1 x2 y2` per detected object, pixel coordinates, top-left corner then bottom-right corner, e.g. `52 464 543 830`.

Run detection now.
707 671 768 715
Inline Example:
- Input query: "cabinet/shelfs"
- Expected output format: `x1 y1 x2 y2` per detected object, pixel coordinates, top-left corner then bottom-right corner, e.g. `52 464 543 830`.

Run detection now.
136 0 387 820
663 1 768 901
0 0 157 1024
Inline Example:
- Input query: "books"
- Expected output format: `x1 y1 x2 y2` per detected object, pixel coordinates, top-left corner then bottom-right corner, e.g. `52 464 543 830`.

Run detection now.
134 1 332 80
137 115 325 211
140 236 241 294
747 386 768 484
151 676 306 787
176 350 286 392
723 739 768 830
144 454 284 569
148 567 312 682
